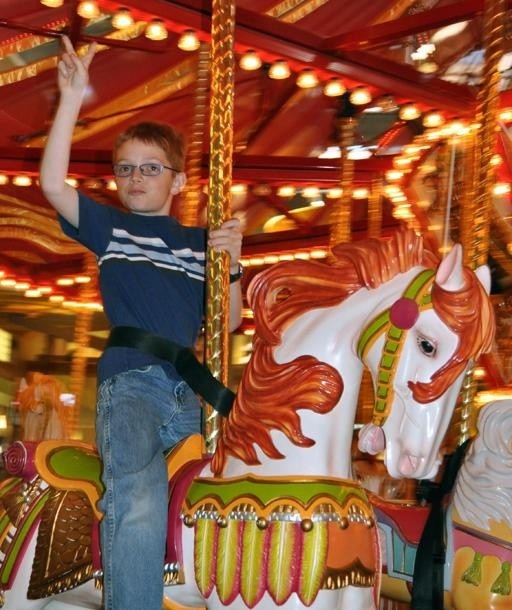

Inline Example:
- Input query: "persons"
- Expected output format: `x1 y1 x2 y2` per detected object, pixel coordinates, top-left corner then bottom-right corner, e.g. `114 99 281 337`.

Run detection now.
40 34 243 610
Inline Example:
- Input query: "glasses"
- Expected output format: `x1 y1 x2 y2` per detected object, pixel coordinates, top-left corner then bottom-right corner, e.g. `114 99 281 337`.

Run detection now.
111 162 179 178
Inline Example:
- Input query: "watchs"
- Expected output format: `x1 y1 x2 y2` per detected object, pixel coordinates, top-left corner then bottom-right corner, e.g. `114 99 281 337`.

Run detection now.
229 263 243 283
